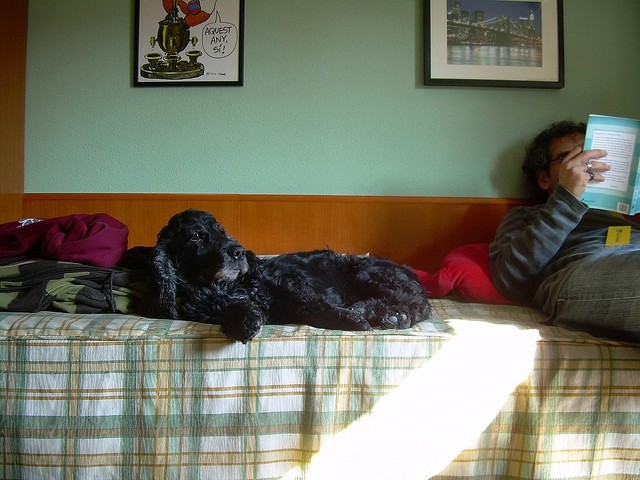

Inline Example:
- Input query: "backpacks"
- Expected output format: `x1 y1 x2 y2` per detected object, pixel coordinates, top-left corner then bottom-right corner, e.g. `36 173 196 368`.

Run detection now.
1 246 161 316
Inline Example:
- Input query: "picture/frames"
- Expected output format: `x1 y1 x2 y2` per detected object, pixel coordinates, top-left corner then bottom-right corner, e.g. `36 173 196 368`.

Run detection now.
131 0 246 88
421 1 567 91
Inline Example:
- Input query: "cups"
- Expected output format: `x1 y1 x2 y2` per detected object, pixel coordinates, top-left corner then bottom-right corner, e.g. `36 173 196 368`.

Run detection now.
163 55 181 70
144 53 162 70
185 50 202 66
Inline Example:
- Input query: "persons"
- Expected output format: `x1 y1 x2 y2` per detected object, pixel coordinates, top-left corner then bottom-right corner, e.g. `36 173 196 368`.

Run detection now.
488 119 640 337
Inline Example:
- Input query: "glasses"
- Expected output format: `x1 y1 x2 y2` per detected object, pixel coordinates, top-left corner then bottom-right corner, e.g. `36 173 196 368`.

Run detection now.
540 151 570 173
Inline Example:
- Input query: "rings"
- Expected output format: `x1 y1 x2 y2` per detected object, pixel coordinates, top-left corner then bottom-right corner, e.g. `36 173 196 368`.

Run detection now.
585 162 592 173
589 171 594 181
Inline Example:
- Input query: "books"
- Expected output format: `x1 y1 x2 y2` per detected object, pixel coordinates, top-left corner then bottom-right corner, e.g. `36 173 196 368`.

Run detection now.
574 114 640 216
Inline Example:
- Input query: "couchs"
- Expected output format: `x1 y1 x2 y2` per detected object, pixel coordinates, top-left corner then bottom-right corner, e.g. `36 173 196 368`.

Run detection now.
2 293 640 478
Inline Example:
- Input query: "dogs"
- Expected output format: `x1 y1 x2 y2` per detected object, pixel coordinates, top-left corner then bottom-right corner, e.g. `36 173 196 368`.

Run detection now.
143 208 433 345
